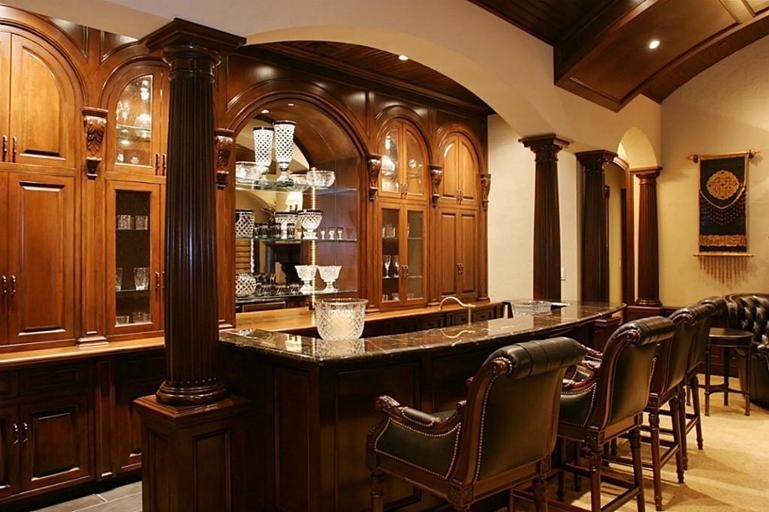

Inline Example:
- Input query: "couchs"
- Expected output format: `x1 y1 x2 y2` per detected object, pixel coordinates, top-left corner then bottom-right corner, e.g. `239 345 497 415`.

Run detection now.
724 294 769 408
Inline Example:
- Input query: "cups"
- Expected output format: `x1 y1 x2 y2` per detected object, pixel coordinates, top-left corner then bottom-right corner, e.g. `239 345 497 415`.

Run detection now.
234 208 256 239
313 298 369 341
133 267 149 291
135 215 148 230
392 293 399 300
117 214 131 230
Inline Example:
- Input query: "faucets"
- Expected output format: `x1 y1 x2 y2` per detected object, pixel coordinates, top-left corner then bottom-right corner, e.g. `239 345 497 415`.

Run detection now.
439 327 476 339
438 295 473 323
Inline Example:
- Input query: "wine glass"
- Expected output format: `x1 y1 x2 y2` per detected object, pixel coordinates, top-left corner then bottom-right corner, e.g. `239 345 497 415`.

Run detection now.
252 126 275 186
295 265 318 292
320 227 344 240
383 255 392 278
273 211 298 240
392 255 399 278
318 266 342 293
271 119 297 186
297 209 323 240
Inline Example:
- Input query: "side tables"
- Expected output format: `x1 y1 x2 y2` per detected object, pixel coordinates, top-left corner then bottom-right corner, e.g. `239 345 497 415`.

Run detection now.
687 327 754 416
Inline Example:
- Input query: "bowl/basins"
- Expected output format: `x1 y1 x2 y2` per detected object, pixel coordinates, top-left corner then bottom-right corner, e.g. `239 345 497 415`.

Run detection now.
306 170 336 190
235 160 265 184
